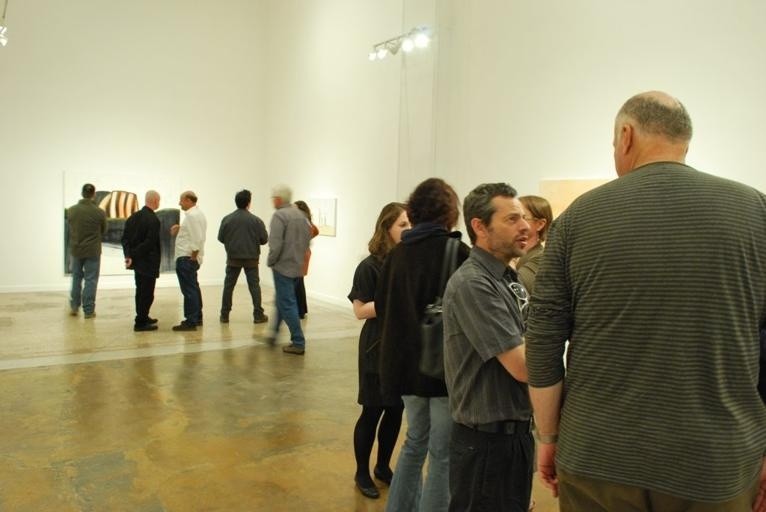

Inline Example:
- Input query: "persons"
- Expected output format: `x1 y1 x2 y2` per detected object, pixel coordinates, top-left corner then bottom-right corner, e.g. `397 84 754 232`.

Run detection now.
252 184 305 354
121 190 161 332
506 195 554 472
170 191 207 331
441 182 535 512
292 201 318 317
374 177 472 512
523 92 766 512
348 201 413 499
67 184 108 320
218 190 269 323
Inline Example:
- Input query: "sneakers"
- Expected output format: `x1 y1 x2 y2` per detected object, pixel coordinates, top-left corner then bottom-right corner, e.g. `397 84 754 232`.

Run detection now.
220 316 229 323
69 310 95 319
283 345 304 355
254 315 268 323
253 333 275 346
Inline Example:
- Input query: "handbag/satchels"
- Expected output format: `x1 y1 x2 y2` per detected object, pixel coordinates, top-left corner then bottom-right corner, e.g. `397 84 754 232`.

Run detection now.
420 297 444 378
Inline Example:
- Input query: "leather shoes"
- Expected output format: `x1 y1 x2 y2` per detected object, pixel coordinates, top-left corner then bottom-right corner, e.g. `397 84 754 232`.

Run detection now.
134 317 158 331
173 320 202 331
355 473 379 498
374 464 393 485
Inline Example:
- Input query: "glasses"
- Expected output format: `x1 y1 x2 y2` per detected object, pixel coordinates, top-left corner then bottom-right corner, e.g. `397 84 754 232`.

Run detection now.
508 281 529 323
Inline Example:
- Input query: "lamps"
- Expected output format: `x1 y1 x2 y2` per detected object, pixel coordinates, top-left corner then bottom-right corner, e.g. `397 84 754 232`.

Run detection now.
367 26 432 61
0 16 9 47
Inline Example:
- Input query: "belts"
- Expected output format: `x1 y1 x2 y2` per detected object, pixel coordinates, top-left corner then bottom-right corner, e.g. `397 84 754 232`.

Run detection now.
463 415 535 435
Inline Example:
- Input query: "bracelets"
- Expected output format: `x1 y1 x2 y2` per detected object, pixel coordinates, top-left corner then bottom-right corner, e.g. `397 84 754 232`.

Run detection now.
537 430 558 445
